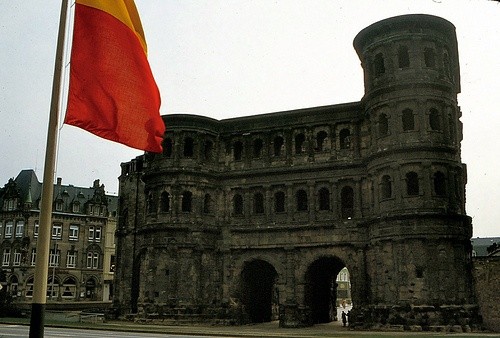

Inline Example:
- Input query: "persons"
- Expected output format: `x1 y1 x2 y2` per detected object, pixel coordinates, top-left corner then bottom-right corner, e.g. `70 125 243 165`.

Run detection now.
346 310 351 327
341 311 347 327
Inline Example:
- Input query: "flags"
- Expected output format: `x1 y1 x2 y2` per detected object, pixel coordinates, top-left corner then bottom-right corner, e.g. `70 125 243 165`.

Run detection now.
63 1 165 152
